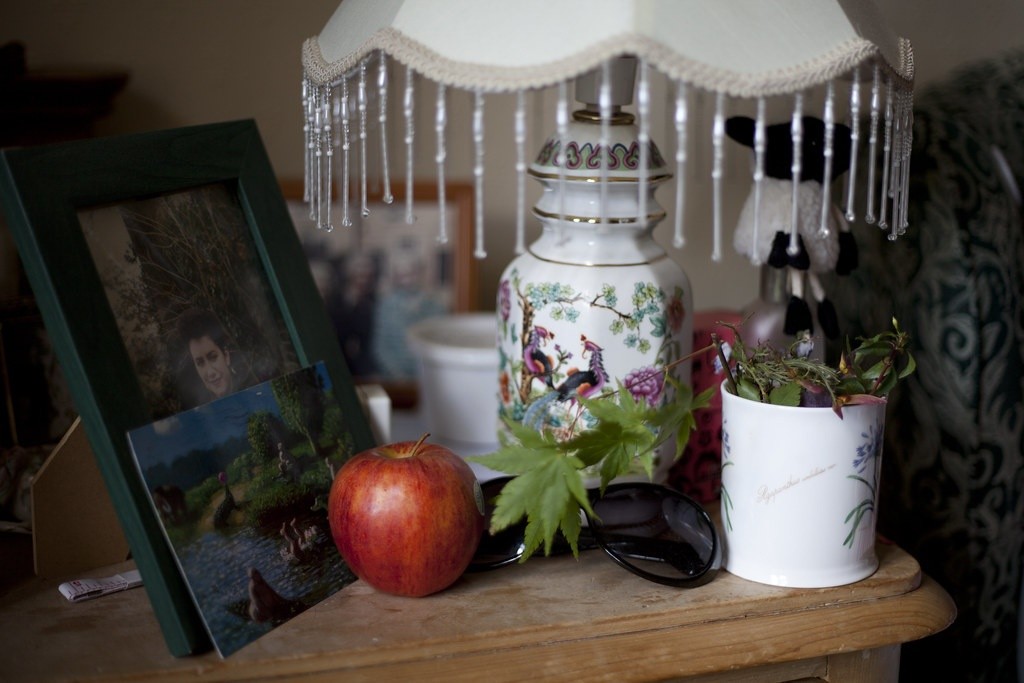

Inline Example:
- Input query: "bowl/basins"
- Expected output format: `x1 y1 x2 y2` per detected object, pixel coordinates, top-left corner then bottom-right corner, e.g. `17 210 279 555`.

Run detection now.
401 314 502 448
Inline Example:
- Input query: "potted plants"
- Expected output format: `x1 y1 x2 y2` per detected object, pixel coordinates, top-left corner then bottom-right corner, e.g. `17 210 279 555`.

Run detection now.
467 311 917 592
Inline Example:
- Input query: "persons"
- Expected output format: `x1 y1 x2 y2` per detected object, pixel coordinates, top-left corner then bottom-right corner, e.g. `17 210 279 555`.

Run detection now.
153 486 186 522
277 442 296 470
280 517 307 559
248 566 285 623
185 318 269 404
301 224 451 377
325 456 338 475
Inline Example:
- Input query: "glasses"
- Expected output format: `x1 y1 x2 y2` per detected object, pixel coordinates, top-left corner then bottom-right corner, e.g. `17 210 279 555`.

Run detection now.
469 475 718 589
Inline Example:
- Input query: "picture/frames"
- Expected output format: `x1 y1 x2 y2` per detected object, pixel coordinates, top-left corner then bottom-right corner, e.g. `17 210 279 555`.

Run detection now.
282 173 481 414
2 115 387 658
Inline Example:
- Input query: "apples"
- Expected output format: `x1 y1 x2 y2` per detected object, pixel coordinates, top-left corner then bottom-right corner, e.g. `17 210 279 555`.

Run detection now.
328 440 484 598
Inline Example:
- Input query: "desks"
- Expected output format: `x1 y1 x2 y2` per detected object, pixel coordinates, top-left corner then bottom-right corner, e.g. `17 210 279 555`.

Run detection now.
0 405 960 682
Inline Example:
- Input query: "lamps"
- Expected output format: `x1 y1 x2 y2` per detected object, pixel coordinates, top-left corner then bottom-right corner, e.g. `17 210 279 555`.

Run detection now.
299 0 921 530
